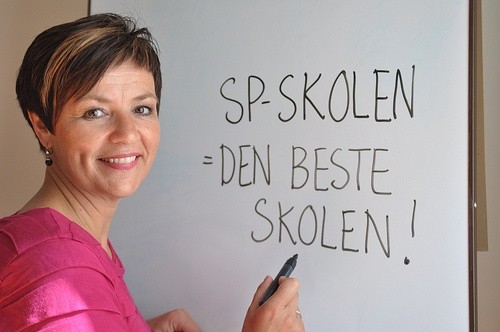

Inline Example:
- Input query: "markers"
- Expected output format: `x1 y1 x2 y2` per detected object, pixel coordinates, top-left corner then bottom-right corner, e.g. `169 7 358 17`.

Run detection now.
259 253 299 306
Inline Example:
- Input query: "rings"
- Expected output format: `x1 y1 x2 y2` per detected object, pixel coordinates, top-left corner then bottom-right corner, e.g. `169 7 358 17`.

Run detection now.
295 309 303 320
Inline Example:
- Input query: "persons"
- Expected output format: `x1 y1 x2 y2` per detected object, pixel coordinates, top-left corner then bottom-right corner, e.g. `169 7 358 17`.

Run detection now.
0 11 307 332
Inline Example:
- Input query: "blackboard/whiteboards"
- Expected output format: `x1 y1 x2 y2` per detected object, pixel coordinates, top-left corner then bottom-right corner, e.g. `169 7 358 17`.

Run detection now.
86 0 477 330
